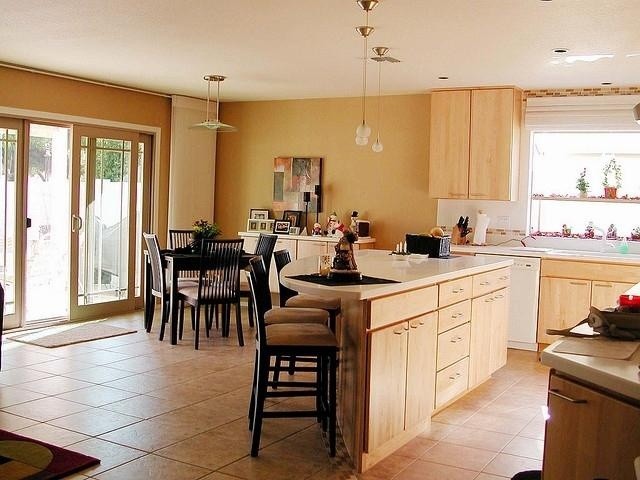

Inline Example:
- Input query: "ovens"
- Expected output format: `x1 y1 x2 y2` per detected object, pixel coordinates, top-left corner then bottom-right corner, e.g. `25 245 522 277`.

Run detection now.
475 254 542 344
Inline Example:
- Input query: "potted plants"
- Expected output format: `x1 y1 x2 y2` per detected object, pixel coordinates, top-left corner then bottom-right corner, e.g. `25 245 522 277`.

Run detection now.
575 168 591 199
585 222 596 240
605 224 619 240
563 223 571 237
602 158 622 199
631 227 639 240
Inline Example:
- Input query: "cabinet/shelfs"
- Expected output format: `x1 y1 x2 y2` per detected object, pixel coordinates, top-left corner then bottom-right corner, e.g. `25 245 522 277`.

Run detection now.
359 265 509 475
538 259 639 351
431 91 520 202
541 367 640 480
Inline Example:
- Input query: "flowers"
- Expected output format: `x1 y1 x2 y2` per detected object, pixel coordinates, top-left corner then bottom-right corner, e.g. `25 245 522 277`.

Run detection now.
191 220 222 252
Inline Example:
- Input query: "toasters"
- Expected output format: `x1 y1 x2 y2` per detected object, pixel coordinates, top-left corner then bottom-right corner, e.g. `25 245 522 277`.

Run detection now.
405 233 450 258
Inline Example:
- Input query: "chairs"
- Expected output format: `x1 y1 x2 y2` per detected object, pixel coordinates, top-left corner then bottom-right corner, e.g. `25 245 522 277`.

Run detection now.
248 249 342 457
141 230 272 350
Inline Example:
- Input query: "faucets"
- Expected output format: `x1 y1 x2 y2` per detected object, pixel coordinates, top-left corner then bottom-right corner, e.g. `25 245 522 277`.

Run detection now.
592 224 615 253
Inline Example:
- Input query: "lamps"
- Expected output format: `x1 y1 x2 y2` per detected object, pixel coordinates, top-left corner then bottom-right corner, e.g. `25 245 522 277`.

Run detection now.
188 76 238 131
354 0 388 153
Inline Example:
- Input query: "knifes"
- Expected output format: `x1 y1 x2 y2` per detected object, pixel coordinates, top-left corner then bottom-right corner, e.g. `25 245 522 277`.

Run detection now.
457 215 472 236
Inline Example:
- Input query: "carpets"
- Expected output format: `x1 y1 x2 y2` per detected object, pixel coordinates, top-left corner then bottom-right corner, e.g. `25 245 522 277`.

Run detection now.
2 432 99 480
7 322 137 349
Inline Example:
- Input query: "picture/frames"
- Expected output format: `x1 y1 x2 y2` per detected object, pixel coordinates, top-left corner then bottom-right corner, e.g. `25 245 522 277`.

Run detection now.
247 209 301 235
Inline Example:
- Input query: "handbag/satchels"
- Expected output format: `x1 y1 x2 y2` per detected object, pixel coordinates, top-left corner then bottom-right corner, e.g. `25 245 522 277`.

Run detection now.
543 308 640 341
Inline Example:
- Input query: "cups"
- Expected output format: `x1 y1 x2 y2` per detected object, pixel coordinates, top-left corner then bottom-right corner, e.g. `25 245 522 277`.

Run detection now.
318 255 331 276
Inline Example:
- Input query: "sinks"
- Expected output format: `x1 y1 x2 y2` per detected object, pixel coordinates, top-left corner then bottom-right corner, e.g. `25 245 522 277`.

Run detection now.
553 250 640 260
